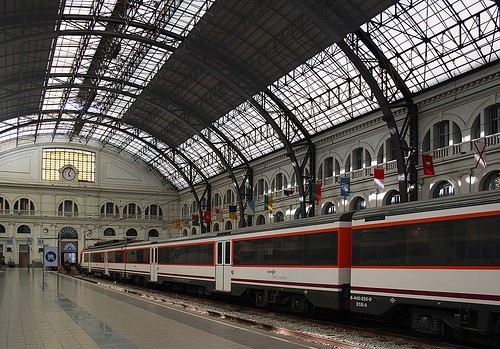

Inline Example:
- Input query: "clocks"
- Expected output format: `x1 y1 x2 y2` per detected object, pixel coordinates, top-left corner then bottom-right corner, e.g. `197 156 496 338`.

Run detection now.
62 166 76 180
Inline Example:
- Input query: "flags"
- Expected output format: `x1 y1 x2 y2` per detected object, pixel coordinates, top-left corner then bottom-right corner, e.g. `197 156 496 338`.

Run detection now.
422 154 435 176
215 208 224 222
181 215 189 228
373 168 385 189
311 183 321 201
204 211 211 222
340 177 351 196
264 195 272 211
191 214 199 226
162 220 167 230
473 141 487 170
229 205 237 220
173 218 180 230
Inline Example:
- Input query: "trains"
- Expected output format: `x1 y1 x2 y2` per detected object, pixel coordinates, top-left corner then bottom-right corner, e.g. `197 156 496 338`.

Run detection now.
80 190 499 348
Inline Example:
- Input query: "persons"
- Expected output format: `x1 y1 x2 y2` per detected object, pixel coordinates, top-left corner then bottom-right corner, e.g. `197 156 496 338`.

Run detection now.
63 258 71 274
